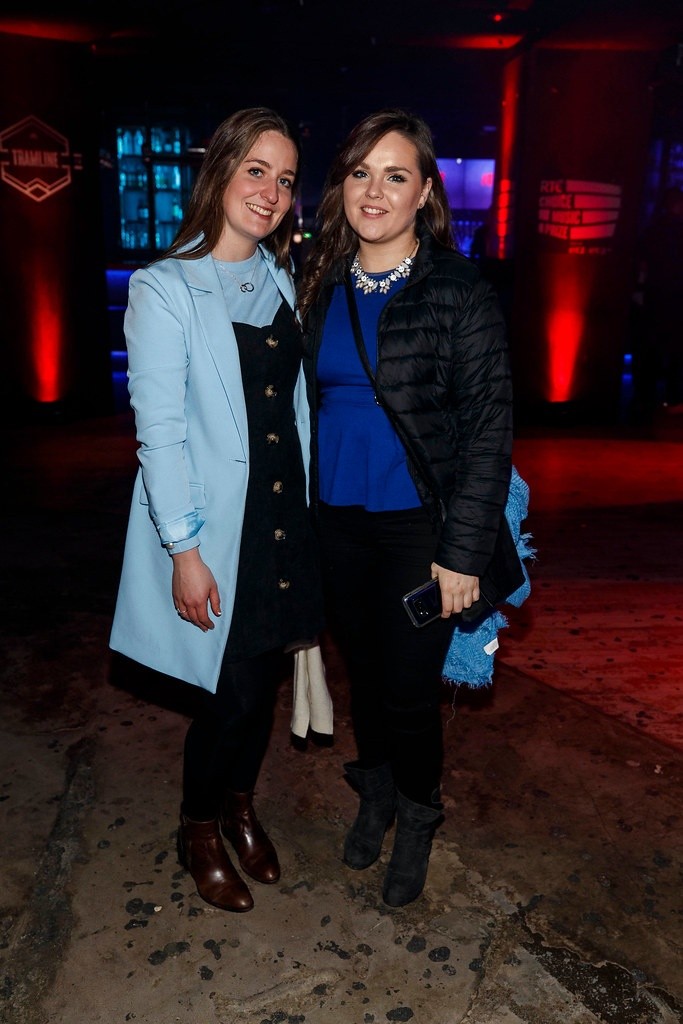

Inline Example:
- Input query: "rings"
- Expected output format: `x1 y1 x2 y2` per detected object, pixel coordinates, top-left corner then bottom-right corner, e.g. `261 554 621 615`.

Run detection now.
177 607 188 615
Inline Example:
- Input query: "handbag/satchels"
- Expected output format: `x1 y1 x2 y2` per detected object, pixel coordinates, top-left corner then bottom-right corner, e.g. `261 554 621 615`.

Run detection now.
457 513 527 632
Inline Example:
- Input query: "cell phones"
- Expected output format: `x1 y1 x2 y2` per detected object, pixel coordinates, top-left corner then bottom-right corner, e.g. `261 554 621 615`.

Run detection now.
403 576 443 628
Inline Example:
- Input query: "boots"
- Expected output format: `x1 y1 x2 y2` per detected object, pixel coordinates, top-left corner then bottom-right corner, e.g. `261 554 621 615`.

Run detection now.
217 786 282 886
176 813 255 914
382 790 444 907
340 757 397 870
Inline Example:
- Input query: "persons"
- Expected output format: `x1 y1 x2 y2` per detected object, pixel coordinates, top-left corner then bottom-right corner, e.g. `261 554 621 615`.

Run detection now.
307 111 526 907
105 108 315 912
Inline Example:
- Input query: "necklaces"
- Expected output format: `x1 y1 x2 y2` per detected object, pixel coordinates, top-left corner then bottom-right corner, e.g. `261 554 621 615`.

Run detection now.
213 256 260 294
351 237 420 294
